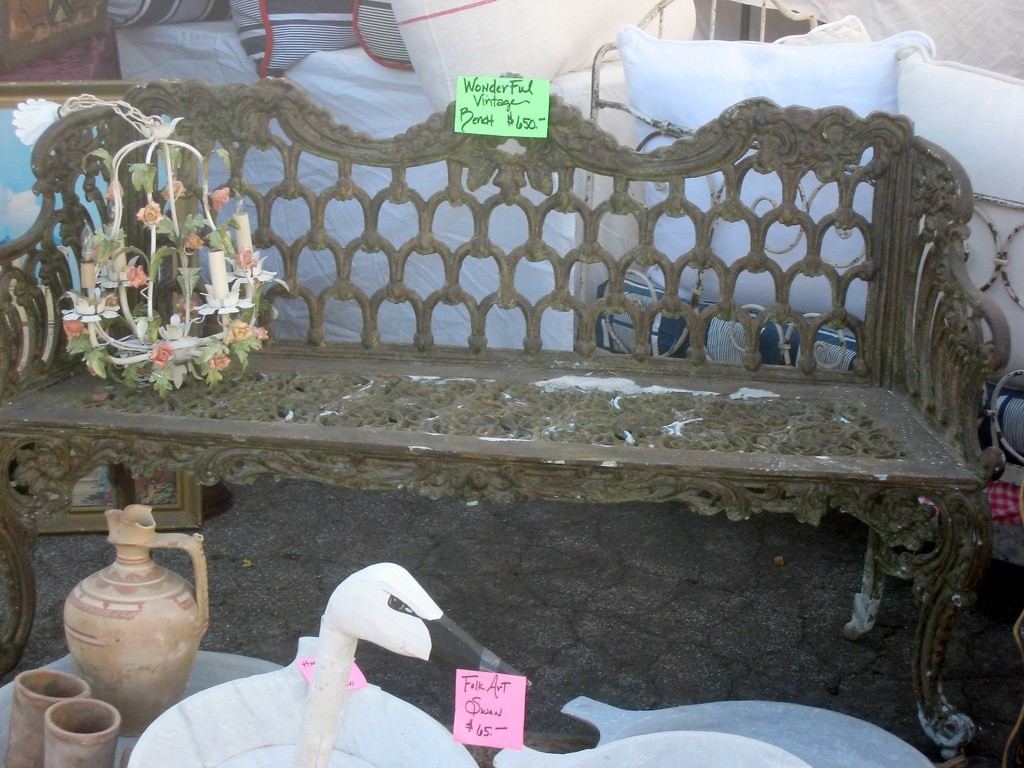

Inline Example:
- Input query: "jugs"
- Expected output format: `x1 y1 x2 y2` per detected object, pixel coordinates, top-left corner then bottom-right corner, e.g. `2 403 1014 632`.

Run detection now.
62 503 210 737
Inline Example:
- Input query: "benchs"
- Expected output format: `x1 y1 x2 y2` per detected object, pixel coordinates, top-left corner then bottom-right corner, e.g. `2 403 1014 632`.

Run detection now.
0 76 1012 759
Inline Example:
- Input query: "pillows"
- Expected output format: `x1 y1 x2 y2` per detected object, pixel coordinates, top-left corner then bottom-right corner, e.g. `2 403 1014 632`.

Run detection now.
0 0 1024 466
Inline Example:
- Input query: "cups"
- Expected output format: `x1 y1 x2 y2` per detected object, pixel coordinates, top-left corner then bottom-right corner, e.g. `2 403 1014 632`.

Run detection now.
2 668 92 767
44 698 122 768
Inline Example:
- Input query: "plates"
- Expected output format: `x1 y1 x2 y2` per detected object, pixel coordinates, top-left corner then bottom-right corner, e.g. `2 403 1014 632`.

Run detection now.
127 637 482 768
560 695 936 768
491 731 814 768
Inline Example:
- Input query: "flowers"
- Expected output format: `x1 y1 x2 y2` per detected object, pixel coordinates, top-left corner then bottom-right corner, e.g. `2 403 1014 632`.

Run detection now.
57 147 290 398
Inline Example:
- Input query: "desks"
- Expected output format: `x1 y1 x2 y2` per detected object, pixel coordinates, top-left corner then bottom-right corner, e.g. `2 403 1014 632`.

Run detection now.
0 651 285 768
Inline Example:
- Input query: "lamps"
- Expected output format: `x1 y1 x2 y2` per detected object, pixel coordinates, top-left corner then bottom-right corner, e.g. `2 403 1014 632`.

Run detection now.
14 92 290 398
